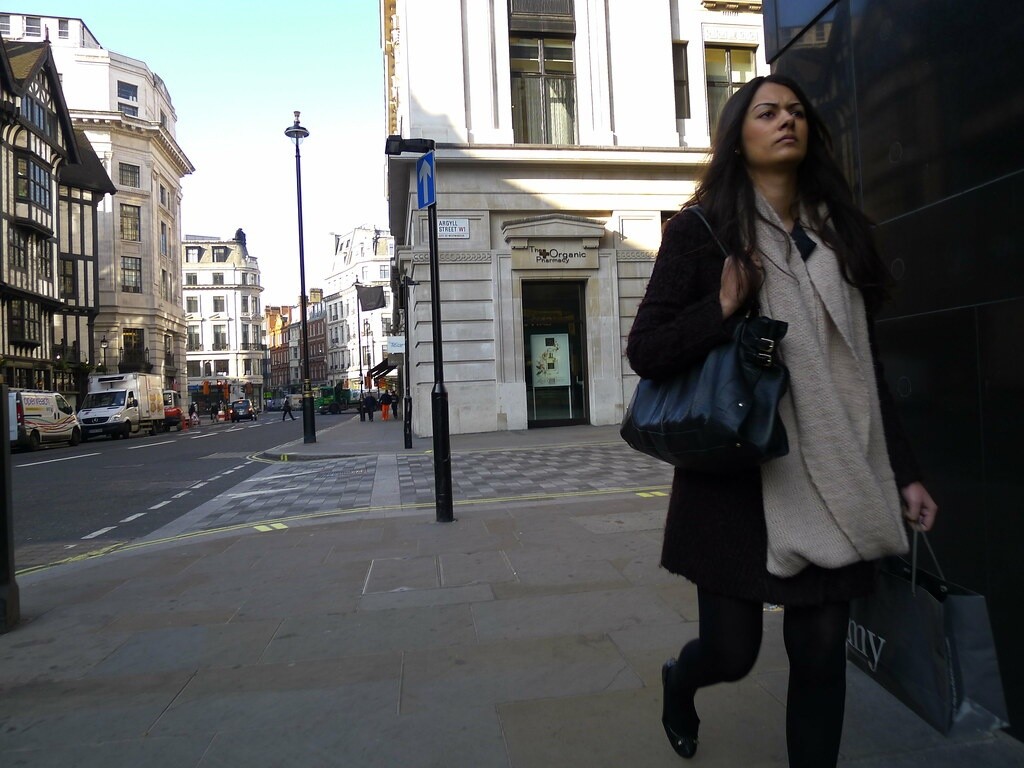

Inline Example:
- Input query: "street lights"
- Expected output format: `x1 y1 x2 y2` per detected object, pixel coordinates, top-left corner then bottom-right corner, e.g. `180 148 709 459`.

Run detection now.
283 111 318 444
400 274 421 447
100 335 108 375
353 278 365 422
385 135 453 521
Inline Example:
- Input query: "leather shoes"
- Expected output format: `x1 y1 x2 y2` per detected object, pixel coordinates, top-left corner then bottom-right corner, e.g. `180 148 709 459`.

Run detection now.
662 660 704 760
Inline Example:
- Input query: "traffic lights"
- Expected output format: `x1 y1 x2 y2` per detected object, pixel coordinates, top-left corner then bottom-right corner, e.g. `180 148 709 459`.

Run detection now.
217 381 222 392
240 385 245 393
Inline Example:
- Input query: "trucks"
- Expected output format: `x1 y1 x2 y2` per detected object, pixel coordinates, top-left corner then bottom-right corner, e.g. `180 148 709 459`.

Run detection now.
76 372 165 440
282 394 303 410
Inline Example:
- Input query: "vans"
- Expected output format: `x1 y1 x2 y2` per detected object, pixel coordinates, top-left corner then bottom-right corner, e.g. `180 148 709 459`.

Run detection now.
8 387 81 450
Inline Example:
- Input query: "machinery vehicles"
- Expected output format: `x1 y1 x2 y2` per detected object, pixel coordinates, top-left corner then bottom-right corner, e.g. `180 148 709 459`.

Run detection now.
155 390 184 431
314 387 351 414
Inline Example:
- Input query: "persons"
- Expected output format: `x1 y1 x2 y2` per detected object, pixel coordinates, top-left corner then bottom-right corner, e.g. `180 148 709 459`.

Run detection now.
391 391 399 419
364 393 378 422
377 390 392 421
282 396 297 421
627 74 937 768
210 404 219 424
188 402 199 428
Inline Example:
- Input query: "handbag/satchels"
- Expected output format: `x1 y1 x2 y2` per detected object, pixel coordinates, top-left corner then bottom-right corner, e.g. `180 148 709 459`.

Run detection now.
847 522 1012 740
617 312 790 468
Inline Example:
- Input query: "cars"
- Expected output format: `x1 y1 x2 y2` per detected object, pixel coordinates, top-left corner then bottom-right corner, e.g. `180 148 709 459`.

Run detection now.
230 399 257 423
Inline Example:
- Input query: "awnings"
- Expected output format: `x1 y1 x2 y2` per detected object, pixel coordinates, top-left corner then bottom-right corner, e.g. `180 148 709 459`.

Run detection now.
367 358 398 382
